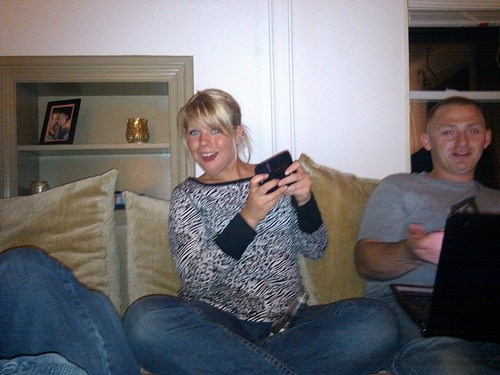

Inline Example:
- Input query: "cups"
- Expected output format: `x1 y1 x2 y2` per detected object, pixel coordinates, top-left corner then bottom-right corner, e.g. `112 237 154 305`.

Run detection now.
125 116 149 143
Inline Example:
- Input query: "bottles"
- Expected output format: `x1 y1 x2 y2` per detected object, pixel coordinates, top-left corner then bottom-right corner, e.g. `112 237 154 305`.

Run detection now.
32 181 49 195
114 190 124 209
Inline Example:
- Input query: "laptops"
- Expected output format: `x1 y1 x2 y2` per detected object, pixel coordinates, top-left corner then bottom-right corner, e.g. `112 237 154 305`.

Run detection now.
390 212 500 342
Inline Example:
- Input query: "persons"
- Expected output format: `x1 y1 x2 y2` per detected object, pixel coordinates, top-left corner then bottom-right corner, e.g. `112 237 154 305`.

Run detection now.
353 96 500 375
122 88 401 375
45 108 71 141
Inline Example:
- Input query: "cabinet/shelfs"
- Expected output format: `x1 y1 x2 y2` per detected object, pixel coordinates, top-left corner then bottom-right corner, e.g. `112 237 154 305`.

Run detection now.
0 56 194 225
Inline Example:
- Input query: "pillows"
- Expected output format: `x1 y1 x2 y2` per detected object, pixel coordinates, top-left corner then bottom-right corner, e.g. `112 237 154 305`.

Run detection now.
122 190 177 305
298 153 379 306
0 169 120 310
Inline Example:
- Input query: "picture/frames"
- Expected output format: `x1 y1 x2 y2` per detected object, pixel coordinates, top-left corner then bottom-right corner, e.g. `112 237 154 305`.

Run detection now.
39 98 81 145
